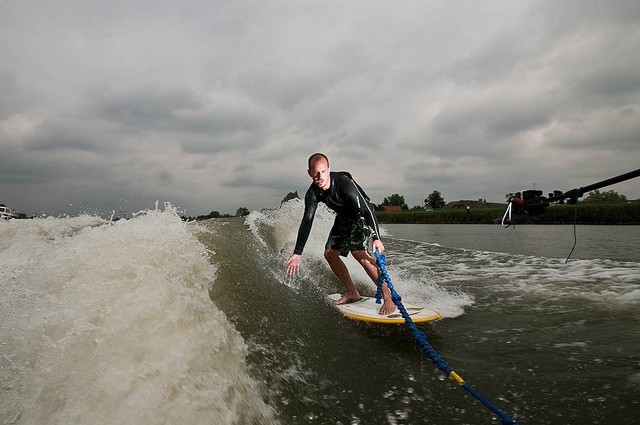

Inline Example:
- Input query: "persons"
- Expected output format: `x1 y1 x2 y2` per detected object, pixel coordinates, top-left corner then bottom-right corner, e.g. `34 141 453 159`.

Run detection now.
285 152 397 315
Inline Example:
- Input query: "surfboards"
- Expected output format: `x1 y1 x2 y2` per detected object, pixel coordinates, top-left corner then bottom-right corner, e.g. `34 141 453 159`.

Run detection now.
328 292 443 324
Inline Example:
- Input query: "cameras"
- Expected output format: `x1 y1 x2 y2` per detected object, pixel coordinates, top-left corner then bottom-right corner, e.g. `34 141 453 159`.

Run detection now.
505 190 551 224
